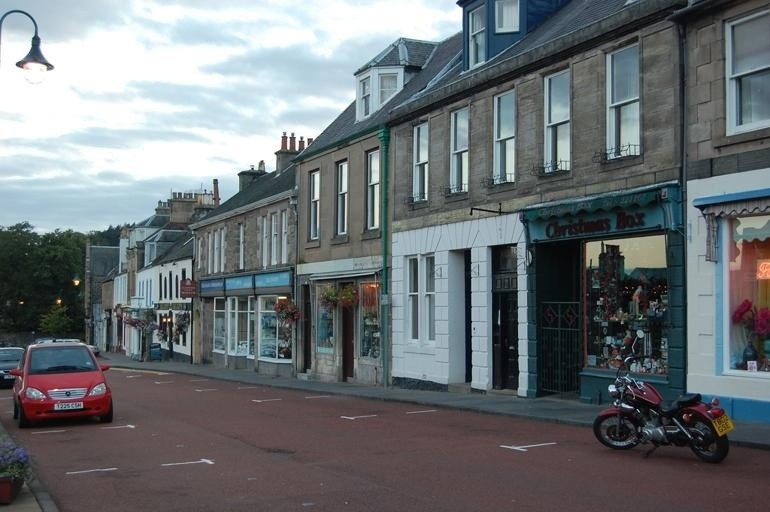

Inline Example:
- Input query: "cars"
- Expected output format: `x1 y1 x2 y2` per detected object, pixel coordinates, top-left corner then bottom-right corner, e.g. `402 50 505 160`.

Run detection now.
8 338 115 429
0 337 101 388
213 335 292 360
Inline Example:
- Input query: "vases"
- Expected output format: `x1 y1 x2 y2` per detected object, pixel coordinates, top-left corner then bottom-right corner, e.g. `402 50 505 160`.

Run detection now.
1 477 26 507
743 341 758 364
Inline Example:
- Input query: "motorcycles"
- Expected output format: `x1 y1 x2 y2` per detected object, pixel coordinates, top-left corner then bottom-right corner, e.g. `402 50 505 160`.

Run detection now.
594 329 735 464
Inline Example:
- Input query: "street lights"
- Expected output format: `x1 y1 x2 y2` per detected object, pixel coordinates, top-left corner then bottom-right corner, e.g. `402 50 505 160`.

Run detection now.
0 10 56 74
72 264 95 345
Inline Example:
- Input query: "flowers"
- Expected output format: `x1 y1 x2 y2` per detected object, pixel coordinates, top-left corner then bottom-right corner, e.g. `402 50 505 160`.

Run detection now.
336 282 361 309
275 296 304 325
317 284 339 307
1 437 38 479
732 299 770 345
